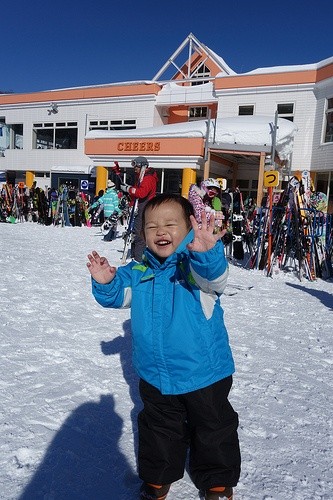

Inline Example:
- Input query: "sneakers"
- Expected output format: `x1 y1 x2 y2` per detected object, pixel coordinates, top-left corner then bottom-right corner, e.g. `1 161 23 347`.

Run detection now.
205 486 233 500
141 480 170 500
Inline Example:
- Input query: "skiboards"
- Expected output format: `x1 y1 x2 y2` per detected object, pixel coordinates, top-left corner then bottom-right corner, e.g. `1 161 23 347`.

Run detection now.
0 181 48 225
120 166 146 264
243 170 333 281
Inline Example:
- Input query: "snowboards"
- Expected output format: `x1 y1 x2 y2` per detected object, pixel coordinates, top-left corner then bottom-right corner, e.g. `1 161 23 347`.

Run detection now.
100 193 133 235
46 182 94 226
209 190 251 260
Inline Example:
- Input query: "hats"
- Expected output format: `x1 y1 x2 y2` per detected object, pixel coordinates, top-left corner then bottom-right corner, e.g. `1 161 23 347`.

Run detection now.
107 180 115 187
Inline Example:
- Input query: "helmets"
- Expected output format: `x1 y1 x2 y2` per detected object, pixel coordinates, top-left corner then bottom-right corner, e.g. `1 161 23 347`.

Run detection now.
203 177 221 190
134 157 148 168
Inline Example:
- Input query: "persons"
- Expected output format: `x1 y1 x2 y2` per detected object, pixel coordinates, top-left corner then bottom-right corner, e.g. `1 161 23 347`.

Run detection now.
98 180 120 241
87 194 241 500
95 189 104 224
216 178 232 210
120 157 158 263
68 190 76 200
50 188 57 201
190 179 224 229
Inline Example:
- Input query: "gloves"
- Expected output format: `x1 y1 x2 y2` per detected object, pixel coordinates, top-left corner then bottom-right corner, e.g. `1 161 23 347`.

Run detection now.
120 183 132 192
